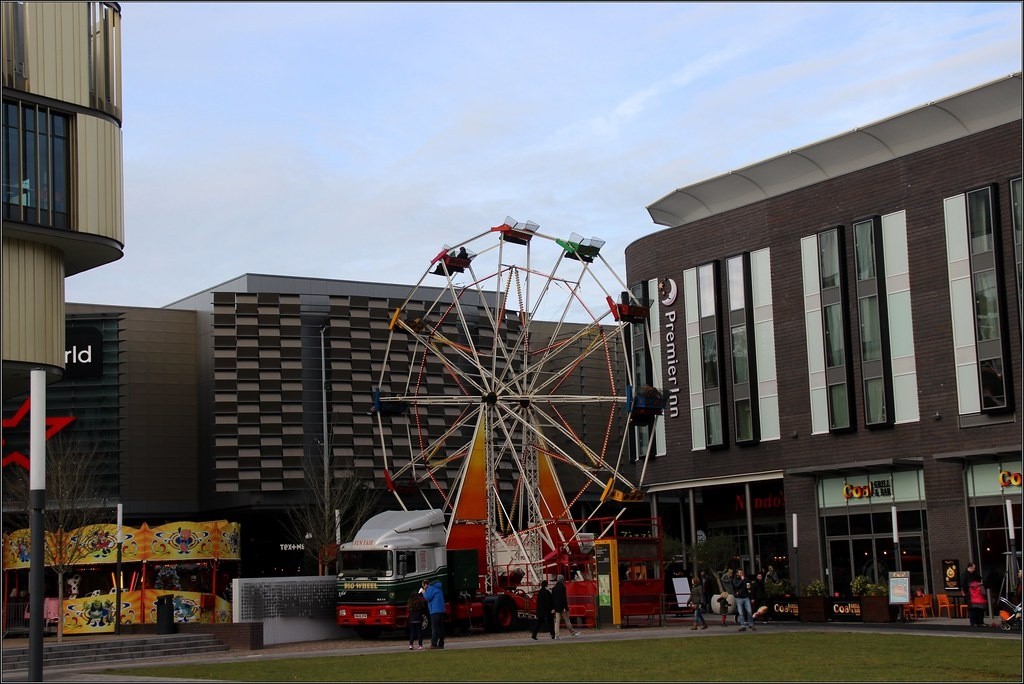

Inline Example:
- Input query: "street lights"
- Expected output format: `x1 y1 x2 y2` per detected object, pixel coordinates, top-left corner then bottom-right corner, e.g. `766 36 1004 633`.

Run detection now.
892 507 900 573
320 321 332 576
792 513 799 598
335 509 341 574
1005 500 1018 596
28 366 47 684
113 503 124 634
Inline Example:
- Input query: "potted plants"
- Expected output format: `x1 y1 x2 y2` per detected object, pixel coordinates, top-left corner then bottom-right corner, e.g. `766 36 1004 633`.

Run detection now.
850 576 887 596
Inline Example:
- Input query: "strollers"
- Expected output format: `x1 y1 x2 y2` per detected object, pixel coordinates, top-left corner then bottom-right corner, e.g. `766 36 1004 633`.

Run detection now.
999 596 1022 631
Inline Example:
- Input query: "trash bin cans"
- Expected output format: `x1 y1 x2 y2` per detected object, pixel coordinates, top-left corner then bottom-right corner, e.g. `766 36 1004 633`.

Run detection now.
157 594 174 634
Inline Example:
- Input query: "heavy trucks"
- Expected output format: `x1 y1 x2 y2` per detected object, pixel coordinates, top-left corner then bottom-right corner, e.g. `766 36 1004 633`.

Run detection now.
335 510 518 640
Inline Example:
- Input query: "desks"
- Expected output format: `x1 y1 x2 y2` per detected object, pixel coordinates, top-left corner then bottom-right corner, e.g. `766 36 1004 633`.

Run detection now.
947 595 967 618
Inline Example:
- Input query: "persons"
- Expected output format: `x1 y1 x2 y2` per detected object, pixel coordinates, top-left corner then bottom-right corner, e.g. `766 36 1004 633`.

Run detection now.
721 569 734 595
732 567 757 632
961 562 989 627
686 576 708 630
530 580 555 640
699 570 713 613
967 574 987 628
407 591 430 650
421 580 447 648
457 247 467 258
717 592 732 628
765 565 778 585
1014 570 1023 606
751 572 769 624
550 574 581 640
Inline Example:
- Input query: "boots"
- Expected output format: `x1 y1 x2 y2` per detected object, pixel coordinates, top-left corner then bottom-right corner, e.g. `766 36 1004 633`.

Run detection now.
690 625 697 630
701 624 708 629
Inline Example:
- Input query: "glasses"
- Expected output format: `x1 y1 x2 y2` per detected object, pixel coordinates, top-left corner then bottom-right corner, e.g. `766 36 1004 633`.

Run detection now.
422 586 425 588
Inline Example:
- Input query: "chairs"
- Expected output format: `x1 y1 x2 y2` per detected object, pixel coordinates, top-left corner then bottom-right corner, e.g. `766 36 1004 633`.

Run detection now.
936 593 955 618
901 594 933 622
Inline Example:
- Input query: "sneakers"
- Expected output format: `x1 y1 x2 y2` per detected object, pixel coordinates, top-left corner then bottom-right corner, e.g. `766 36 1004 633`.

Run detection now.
971 623 977 627
426 645 437 649
722 614 769 632
570 631 580 639
409 645 415 650
554 636 563 640
436 645 444 649
417 644 424 650
978 623 988 627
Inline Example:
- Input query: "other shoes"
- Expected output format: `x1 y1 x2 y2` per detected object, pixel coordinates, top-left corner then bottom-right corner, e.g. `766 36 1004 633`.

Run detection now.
531 635 538 640
551 634 556 640
